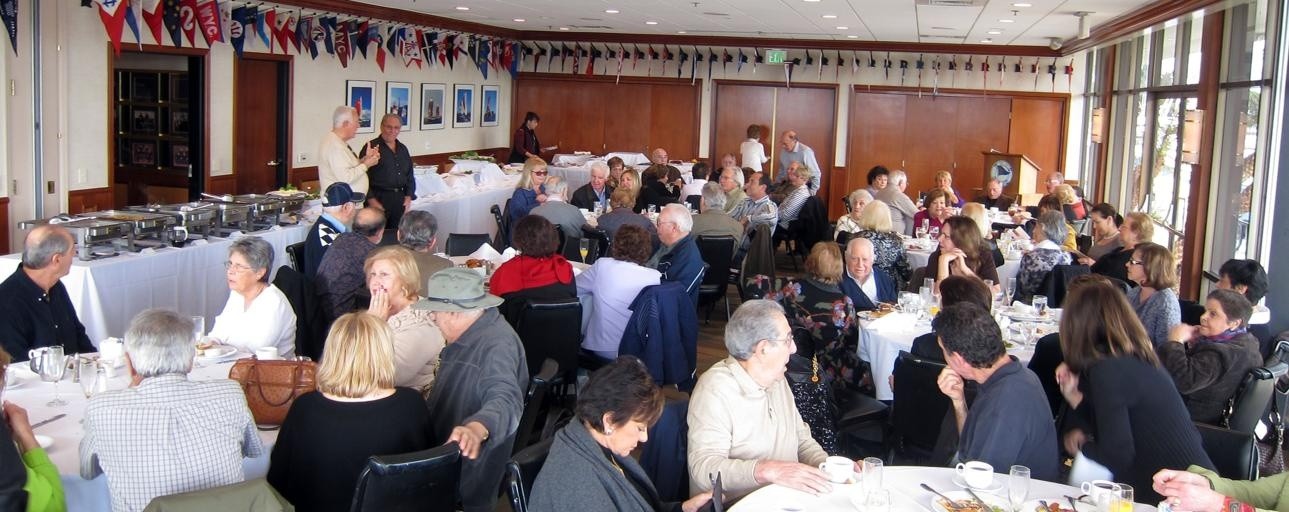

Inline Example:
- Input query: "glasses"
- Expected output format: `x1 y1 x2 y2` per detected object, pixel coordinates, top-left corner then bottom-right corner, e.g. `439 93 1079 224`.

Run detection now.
530 171 549 176
756 332 794 348
1044 179 1058 184
1129 255 1144 266
657 218 673 227
223 260 255 272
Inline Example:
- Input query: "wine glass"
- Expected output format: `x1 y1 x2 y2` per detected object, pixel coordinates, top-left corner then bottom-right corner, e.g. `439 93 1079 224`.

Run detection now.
896 278 1050 353
45 315 206 429
914 221 1000 251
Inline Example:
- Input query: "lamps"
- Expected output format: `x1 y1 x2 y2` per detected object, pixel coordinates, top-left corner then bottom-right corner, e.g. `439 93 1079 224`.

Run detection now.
1175 95 1207 164
1090 106 1106 146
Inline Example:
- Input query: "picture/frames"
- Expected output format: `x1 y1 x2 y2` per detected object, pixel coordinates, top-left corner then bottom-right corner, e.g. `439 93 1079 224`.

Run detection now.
344 77 377 135
452 83 475 128
384 80 412 132
113 67 194 172
480 85 501 127
418 82 447 131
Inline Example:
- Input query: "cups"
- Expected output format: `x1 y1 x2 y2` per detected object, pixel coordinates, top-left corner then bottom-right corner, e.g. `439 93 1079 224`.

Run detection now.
1080 479 1122 503
255 345 280 362
2 370 18 385
1107 482 1134 511
990 206 999 220
203 349 219 356
578 197 692 221
955 459 993 490
1007 465 1030 512
27 346 48 363
819 455 854 484
859 456 884 486
863 488 892 511
1007 203 1019 217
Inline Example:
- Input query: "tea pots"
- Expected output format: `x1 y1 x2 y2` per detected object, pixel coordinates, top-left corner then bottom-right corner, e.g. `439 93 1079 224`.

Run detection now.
29 343 73 383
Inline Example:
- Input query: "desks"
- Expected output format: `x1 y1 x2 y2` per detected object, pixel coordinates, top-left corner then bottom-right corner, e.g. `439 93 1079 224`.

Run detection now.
0 186 323 347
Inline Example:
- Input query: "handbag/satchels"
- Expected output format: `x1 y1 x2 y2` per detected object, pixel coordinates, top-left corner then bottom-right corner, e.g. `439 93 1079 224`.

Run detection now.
1218 366 1285 477
791 354 839 456
228 357 318 431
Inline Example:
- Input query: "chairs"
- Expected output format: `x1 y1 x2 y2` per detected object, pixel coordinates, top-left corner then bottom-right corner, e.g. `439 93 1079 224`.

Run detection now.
282 233 591 512
142 481 296 512
503 146 833 324
829 192 1289 510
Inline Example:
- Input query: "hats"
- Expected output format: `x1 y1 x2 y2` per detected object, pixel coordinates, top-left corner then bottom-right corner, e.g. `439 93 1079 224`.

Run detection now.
409 266 505 313
321 182 366 206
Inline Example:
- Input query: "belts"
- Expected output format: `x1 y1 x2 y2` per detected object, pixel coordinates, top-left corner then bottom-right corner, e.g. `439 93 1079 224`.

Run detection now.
378 186 405 192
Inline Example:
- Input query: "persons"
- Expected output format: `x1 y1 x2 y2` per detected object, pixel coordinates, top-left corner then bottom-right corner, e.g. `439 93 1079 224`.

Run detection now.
305 105 1289 512
0 226 100 355
76 305 263 511
266 312 438 512
0 346 64 511
204 235 299 362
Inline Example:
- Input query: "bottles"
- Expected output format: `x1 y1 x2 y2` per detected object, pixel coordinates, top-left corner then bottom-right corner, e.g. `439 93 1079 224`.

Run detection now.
459 260 491 277
952 206 960 215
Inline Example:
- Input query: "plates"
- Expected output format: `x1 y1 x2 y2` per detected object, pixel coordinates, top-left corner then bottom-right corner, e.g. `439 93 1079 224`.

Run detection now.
193 342 239 360
33 434 55 449
1018 498 1096 512
950 475 999 494
929 490 1011 512
4 377 27 392
856 309 883 321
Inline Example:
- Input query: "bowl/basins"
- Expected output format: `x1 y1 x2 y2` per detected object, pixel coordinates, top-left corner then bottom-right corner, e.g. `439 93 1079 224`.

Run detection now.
1009 249 1022 260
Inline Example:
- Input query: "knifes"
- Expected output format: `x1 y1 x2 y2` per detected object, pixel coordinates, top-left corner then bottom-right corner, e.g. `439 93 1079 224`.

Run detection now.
918 483 964 511
964 487 993 512
30 413 67 432
